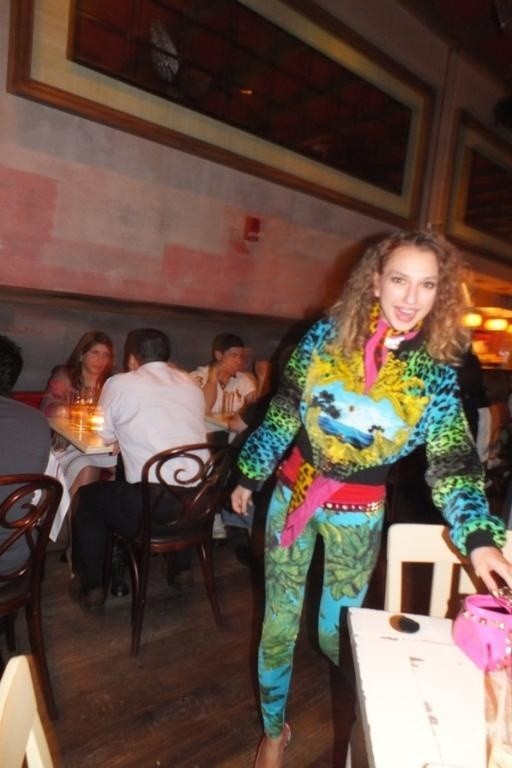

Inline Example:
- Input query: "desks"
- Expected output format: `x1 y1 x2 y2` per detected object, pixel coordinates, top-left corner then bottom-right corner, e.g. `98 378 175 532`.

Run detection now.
341 606 512 768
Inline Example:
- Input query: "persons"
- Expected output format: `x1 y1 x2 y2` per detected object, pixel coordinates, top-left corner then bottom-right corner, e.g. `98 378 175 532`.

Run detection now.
231 229 512 768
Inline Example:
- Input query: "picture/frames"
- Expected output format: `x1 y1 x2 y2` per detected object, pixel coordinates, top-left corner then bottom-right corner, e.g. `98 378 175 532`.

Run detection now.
6 0 438 230
442 106 512 266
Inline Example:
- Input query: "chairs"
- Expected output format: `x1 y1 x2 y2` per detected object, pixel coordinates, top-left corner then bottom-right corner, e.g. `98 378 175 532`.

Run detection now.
0 474 66 768
382 520 512 619
100 442 236 655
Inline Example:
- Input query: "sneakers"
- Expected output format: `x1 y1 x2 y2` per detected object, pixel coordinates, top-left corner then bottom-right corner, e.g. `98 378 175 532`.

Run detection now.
78 588 103 613
254 720 292 767
